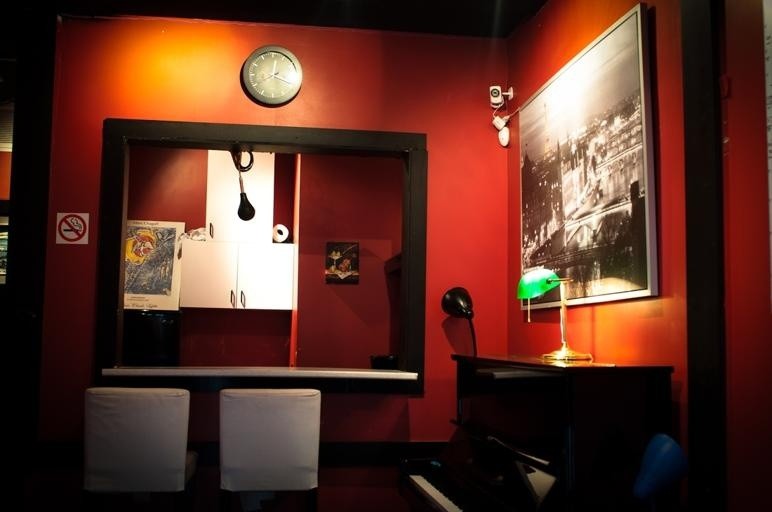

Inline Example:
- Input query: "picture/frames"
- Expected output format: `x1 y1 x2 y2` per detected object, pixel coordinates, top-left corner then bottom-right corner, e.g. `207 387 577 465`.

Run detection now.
519 1 659 312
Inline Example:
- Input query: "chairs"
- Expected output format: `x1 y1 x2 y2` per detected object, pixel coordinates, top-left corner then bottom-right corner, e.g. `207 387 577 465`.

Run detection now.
217 387 322 510
83 387 189 507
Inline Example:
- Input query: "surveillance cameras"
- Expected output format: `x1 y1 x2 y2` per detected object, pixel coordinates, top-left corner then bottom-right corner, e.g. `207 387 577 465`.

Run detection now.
489 86 502 104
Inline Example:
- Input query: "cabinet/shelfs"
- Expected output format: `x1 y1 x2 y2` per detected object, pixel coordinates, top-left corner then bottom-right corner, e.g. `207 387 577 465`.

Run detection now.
449 354 676 512
205 150 275 243
178 242 299 312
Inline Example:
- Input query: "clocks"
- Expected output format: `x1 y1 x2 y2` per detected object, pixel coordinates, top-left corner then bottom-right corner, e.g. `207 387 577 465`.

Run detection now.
240 43 305 107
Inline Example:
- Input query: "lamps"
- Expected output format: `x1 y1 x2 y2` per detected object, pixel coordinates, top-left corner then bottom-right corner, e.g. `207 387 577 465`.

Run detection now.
517 269 592 362
440 283 478 359
